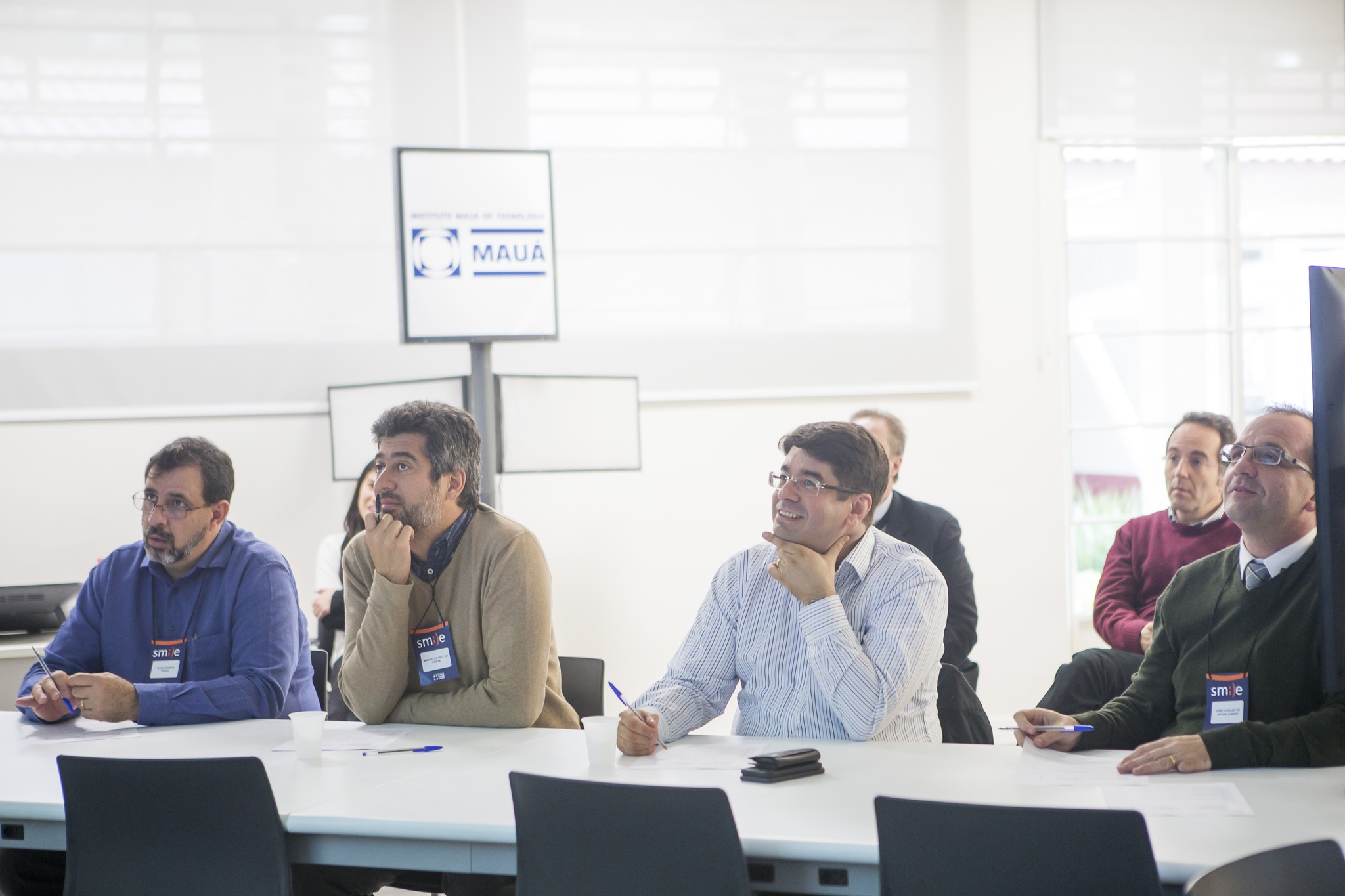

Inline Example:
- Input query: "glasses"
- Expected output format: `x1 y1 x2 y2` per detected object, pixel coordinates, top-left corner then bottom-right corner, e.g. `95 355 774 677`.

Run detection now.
1221 443 1312 473
768 471 862 498
132 490 212 518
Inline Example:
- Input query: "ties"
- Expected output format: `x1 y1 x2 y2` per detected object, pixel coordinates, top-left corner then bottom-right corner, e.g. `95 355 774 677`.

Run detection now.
1245 563 1268 591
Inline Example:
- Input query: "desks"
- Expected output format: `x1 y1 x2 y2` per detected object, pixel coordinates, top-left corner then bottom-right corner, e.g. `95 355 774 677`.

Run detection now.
0 709 1345 896
0 629 58 712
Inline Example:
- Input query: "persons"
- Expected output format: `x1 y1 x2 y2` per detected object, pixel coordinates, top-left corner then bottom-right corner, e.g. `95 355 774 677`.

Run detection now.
16 435 322 726
847 409 980 688
312 400 582 729
1013 409 1344 774
615 418 949 756
1036 410 1241 715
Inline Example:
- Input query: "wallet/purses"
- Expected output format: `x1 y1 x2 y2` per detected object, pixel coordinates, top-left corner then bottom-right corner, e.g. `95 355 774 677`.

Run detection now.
749 748 821 768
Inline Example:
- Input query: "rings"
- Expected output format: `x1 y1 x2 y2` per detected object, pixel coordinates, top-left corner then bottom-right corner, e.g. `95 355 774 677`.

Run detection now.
775 558 781 567
81 699 85 710
1169 754 1176 766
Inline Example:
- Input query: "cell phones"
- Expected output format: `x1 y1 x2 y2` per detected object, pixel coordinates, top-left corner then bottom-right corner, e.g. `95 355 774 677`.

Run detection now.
740 762 824 783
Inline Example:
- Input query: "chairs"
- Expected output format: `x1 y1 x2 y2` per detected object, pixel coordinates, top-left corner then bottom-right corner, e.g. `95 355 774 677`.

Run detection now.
874 796 1164 896
935 662 995 744
509 771 751 896
56 754 293 896
558 657 605 728
1184 838 1345 896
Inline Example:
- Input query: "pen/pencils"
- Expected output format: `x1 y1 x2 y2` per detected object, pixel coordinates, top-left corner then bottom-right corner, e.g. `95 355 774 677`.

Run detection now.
997 725 1094 732
362 745 443 756
31 645 74 712
608 681 668 750
